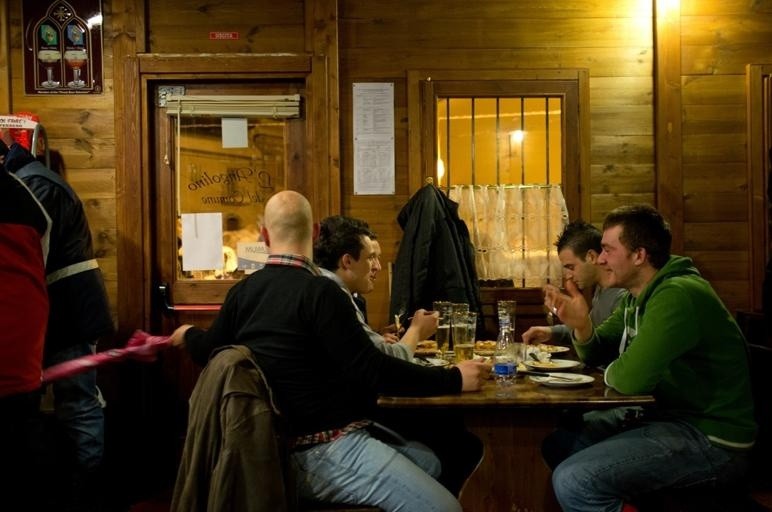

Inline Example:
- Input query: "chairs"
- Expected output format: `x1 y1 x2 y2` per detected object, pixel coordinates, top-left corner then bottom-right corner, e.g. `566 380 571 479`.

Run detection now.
210 346 382 509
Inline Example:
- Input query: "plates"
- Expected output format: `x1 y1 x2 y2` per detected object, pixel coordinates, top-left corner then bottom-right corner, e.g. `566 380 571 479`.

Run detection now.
520 358 581 372
411 357 451 368
519 343 570 354
529 372 596 389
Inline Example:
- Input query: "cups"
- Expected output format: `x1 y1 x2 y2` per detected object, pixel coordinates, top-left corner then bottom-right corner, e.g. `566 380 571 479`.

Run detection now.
497 300 517 338
64 47 88 89
432 301 478 365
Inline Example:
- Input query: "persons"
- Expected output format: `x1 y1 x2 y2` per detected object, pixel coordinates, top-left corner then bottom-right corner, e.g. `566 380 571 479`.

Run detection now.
2 139 123 509
164 190 493 512
351 235 404 344
520 220 627 372
541 200 766 512
312 212 442 363
1 161 58 511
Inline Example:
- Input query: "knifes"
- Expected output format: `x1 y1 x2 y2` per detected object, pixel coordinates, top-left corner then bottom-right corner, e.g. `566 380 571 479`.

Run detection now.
518 370 573 381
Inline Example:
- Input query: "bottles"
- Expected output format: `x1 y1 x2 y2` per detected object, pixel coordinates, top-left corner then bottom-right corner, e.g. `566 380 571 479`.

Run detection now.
494 318 517 400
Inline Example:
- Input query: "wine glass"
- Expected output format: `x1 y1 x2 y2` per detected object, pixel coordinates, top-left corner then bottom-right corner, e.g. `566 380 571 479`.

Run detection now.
37 47 61 89
